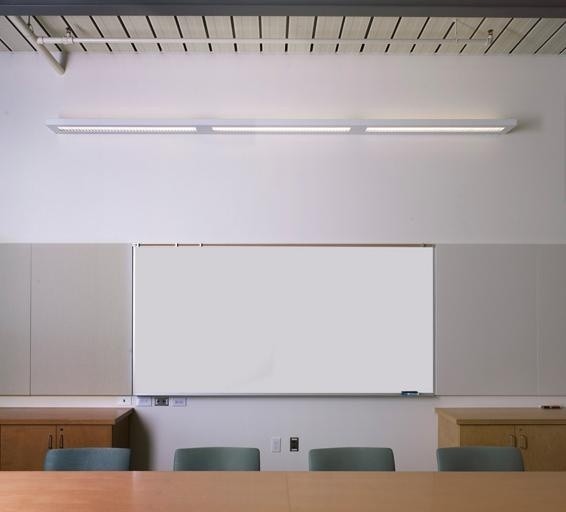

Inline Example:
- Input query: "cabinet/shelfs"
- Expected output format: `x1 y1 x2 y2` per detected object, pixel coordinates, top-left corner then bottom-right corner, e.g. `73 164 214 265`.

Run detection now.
434 406 565 471
1 408 136 471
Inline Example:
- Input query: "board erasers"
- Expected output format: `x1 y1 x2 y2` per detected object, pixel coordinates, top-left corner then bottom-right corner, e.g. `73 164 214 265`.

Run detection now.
402 391 420 396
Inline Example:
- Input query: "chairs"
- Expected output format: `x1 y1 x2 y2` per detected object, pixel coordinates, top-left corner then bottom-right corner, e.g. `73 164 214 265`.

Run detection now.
44 447 135 471
172 447 261 471
436 447 525 471
307 447 397 471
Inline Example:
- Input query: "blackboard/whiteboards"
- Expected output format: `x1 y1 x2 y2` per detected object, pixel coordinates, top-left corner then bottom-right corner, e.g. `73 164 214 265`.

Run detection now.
131 243 436 397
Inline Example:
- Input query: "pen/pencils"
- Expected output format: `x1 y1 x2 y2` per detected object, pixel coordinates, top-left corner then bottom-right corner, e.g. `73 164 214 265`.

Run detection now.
542 405 561 409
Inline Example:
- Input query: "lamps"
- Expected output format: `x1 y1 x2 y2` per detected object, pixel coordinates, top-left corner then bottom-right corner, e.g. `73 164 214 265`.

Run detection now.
46 116 518 136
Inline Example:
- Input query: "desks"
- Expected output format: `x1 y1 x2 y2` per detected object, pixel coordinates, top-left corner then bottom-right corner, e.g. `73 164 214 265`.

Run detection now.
0 470 564 512
